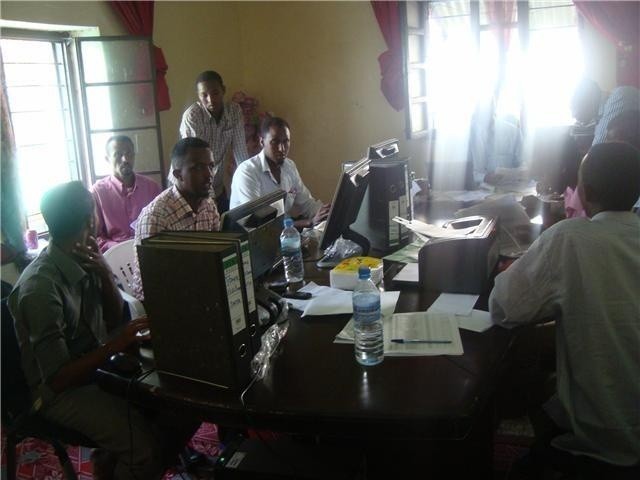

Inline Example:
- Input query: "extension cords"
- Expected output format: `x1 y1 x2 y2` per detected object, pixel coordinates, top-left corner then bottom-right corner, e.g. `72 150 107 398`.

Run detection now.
177 452 218 471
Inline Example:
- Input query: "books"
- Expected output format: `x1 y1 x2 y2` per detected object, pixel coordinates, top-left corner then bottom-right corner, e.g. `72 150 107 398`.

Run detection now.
383 310 464 357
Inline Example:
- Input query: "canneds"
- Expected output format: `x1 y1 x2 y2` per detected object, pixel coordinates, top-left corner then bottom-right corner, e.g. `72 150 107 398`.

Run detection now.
25 229 38 249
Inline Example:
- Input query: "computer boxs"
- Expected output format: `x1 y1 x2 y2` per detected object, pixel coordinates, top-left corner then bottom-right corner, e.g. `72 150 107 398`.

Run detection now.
215 433 369 480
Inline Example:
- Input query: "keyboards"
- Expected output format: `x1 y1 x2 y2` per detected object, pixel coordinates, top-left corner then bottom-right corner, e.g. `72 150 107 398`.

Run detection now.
271 236 310 272
136 328 150 343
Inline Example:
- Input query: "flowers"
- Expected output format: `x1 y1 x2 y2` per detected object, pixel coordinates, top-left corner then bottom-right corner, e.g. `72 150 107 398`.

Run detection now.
0 230 39 273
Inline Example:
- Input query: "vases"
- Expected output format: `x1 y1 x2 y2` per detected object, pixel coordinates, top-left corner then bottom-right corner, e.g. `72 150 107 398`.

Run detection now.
0 261 19 287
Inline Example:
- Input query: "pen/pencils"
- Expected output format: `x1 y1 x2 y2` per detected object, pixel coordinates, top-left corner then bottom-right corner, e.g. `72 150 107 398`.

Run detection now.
391 339 452 344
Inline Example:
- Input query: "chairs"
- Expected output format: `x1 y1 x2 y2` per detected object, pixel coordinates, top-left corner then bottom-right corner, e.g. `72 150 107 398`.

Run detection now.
101 238 149 330
1 280 98 479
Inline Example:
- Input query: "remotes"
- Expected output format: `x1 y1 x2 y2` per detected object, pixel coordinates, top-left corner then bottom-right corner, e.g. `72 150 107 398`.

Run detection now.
286 292 312 300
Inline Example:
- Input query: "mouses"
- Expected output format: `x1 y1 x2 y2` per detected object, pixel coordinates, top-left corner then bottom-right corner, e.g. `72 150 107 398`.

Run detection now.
110 352 141 373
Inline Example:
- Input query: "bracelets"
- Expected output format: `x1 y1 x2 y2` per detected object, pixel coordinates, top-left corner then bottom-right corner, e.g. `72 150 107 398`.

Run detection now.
101 343 115 360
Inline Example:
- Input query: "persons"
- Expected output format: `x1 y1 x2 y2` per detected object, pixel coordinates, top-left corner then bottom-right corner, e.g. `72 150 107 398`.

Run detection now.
133 137 222 303
166 71 249 220
536 152 589 218
7 181 151 480
87 136 162 349
229 118 331 233
489 141 640 480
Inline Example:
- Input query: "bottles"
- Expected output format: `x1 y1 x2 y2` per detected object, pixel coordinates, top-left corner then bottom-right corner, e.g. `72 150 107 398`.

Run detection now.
279 219 305 283
353 266 386 366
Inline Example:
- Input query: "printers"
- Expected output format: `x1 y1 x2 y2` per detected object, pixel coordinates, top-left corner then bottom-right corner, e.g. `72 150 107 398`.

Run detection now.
391 213 502 295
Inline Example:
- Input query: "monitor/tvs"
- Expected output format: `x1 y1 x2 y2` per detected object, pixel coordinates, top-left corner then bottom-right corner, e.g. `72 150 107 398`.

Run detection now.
316 157 371 269
368 138 400 160
219 188 287 330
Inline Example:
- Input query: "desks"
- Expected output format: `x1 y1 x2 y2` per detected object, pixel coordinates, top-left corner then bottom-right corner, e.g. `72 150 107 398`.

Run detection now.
94 201 537 480
